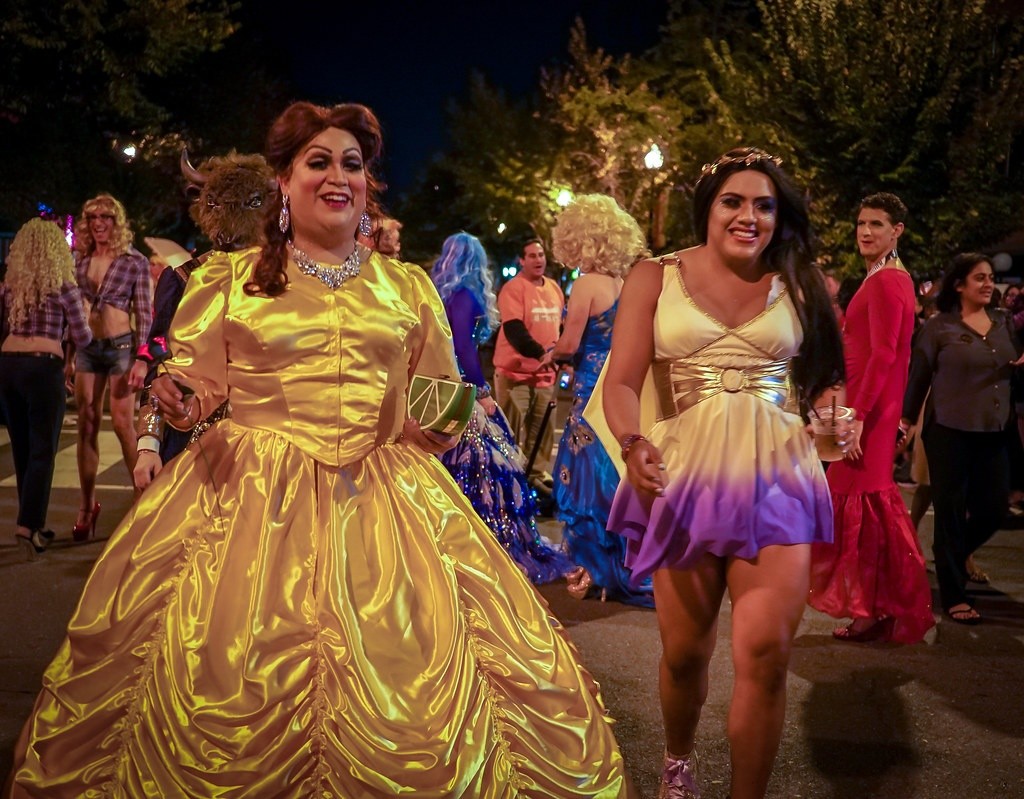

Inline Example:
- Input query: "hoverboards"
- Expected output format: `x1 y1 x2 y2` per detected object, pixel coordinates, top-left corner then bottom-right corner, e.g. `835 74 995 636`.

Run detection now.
524 357 560 519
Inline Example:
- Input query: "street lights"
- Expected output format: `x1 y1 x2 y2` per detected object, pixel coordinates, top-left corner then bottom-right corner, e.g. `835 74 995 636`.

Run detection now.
644 143 664 250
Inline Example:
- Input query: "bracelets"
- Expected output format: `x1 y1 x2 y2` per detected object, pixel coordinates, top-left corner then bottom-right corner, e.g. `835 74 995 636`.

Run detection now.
137 449 151 456
621 433 647 464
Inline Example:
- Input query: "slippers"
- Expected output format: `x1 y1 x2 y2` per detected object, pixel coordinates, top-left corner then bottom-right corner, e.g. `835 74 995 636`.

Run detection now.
831 616 882 642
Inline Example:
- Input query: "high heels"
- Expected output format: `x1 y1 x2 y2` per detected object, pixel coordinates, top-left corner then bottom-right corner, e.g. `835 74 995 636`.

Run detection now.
15 526 56 562
567 571 606 602
72 501 100 540
566 566 585 584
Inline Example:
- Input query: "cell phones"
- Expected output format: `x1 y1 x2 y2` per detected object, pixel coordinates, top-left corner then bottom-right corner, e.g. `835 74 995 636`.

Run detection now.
559 372 570 391
896 426 906 443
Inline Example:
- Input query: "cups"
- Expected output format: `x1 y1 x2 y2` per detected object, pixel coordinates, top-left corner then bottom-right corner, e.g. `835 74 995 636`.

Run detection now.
808 405 851 461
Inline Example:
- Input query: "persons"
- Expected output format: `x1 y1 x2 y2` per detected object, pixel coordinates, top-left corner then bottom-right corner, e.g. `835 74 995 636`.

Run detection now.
0 151 282 560
581 147 856 799
425 233 569 584
8 100 631 797
798 193 1023 643
492 238 564 499
539 193 659 612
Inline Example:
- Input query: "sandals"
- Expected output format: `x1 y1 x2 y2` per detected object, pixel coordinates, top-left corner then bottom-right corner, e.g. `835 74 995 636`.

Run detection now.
967 567 992 584
948 603 980 625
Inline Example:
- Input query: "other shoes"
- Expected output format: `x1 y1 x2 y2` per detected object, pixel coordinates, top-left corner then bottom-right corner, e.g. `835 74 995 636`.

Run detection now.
658 747 699 799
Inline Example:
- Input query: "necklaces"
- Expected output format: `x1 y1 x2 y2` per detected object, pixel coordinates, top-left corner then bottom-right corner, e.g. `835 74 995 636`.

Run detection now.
289 238 361 289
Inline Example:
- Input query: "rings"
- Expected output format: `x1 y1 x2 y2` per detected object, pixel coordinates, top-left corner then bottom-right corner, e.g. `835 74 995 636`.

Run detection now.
149 394 159 412
